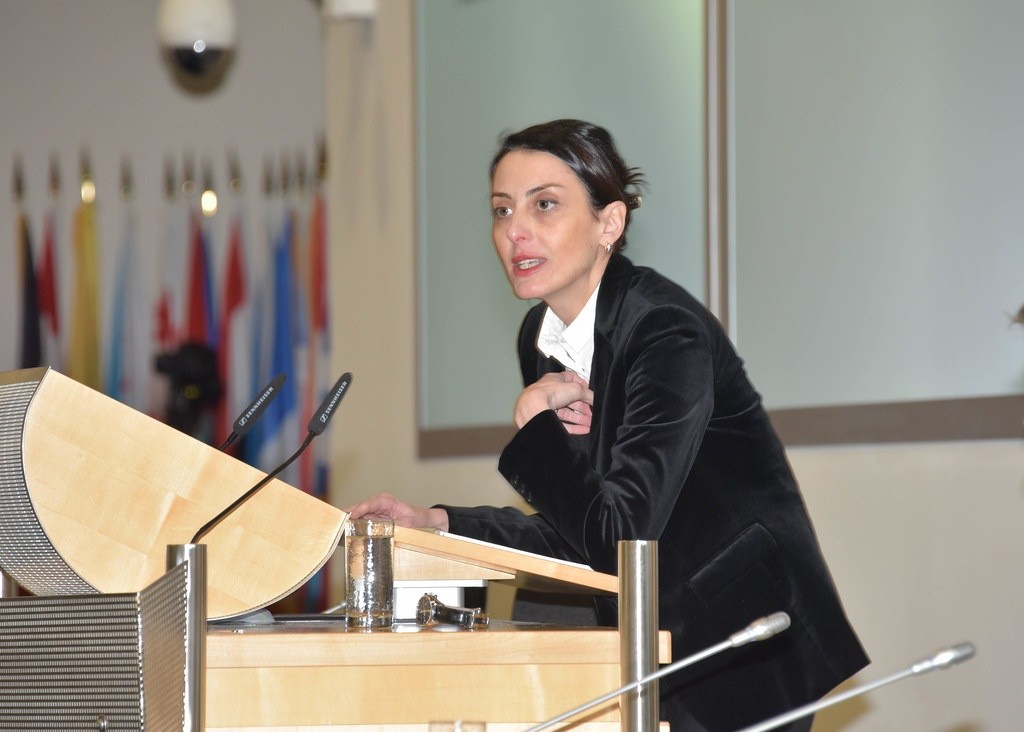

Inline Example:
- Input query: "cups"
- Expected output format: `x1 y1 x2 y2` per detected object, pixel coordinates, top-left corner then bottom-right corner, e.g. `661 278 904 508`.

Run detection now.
344 518 395 634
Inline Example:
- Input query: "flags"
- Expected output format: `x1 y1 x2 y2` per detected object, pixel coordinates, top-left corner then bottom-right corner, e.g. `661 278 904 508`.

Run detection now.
14 132 329 620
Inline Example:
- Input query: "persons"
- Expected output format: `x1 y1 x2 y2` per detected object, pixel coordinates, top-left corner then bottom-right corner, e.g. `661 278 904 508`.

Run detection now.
337 117 867 730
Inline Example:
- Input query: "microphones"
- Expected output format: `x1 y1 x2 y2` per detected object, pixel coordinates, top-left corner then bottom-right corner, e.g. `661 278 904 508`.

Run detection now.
741 644 979 732
187 372 354 543
525 614 793 732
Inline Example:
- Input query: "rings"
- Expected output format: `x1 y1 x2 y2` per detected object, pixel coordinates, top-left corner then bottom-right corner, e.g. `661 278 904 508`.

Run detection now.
554 409 558 415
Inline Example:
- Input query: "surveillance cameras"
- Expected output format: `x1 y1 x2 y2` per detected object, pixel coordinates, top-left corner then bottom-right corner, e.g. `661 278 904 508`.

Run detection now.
155 0 237 93
